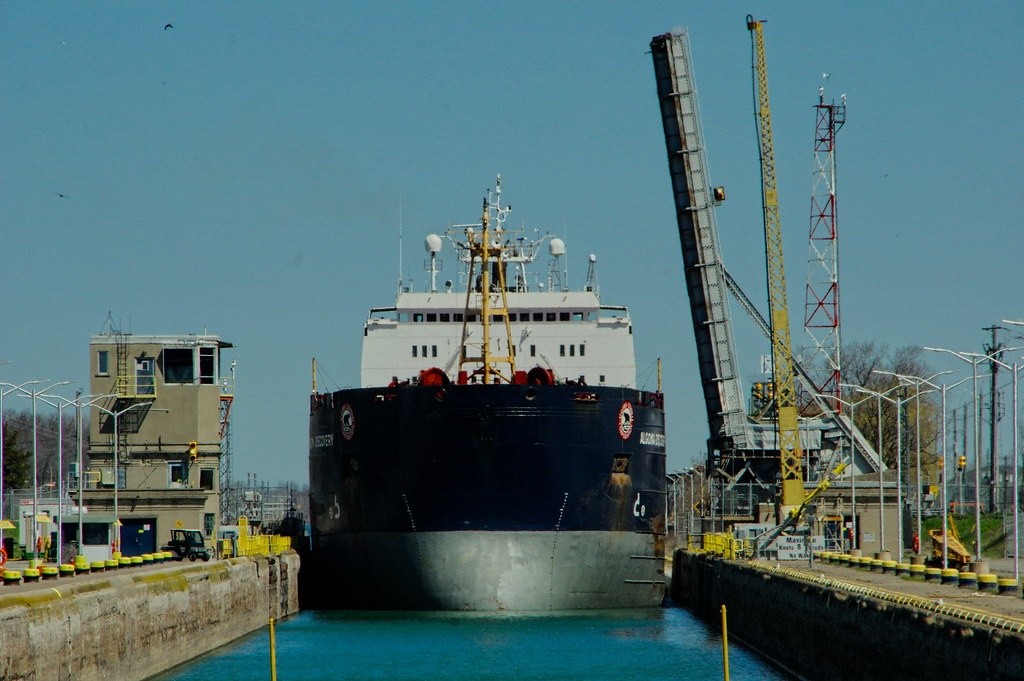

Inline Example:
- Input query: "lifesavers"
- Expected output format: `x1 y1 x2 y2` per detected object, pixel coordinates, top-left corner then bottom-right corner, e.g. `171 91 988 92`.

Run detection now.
0 549 8 566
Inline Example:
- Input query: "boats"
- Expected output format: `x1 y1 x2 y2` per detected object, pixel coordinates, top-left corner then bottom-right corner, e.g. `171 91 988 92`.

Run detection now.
305 170 671 612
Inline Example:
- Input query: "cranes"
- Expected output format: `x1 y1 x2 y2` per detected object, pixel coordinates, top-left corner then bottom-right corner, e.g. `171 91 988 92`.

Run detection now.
741 11 805 526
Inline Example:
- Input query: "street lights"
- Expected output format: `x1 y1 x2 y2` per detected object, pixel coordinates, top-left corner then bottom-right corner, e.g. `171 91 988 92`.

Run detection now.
38 393 123 555
838 382 905 550
855 388 934 563
87 400 154 524
33 391 104 567
794 407 841 486
0 382 71 557
815 393 874 551
872 368 956 555
960 348 1023 581
922 343 1017 563
898 371 984 568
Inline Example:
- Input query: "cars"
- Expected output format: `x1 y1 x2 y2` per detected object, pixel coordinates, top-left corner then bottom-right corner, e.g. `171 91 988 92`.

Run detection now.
160 529 210 562
